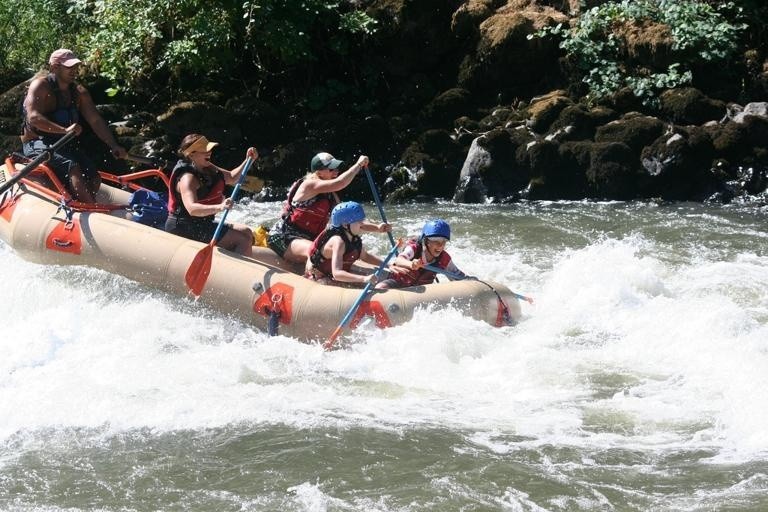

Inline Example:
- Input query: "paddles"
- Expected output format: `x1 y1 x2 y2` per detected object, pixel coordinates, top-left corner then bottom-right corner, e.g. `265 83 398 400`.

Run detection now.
324 239 400 351
185 155 253 295
124 150 266 193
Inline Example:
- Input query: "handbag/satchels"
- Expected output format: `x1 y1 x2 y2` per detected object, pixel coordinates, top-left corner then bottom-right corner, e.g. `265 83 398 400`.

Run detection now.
129 190 168 226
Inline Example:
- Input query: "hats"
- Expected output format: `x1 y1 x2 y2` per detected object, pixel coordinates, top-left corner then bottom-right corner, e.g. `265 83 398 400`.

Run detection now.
49 49 82 68
183 136 219 158
311 152 344 171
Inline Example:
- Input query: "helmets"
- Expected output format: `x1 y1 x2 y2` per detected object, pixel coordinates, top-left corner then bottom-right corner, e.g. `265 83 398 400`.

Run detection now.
421 220 450 240
331 202 364 228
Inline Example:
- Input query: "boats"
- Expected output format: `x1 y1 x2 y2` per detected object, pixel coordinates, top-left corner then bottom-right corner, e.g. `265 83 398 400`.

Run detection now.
0 156 524 350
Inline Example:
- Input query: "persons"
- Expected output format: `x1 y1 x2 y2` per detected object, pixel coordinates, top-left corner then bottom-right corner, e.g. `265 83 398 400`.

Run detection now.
163 133 258 258
375 218 465 292
18 47 127 204
267 152 393 265
304 201 384 290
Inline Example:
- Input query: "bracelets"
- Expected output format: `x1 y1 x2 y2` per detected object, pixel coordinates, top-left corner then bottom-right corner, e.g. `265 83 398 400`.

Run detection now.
357 161 363 170
